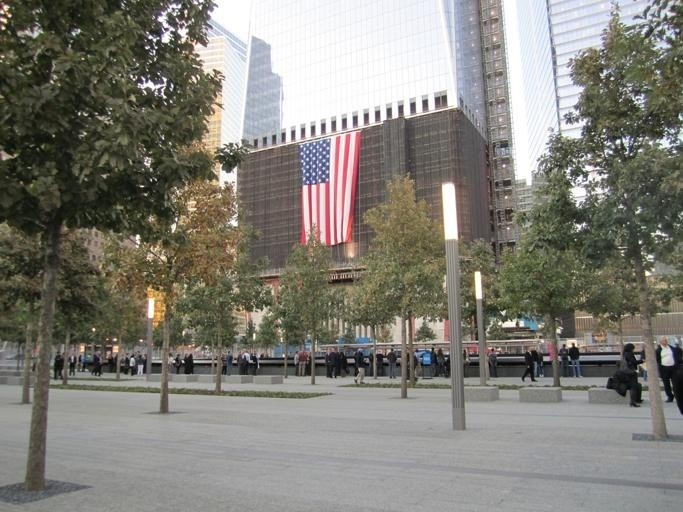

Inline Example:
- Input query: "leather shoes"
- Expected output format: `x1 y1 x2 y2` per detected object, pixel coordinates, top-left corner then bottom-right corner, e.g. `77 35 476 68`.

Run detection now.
629 398 674 407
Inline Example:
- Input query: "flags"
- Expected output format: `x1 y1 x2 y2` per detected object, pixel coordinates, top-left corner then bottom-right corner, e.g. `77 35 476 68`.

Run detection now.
297 130 363 246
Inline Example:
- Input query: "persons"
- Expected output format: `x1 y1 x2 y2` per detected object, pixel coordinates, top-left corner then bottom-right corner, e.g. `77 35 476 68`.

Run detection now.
655 336 679 402
558 344 570 377
52 348 263 381
530 347 538 376
537 349 544 377
294 345 450 385
568 342 583 377
672 365 683 414
640 344 645 360
622 343 646 407
675 342 682 357
489 349 498 376
521 347 536 381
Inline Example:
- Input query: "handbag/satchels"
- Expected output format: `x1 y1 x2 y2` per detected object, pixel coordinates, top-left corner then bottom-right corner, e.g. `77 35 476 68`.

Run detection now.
605 377 620 389
